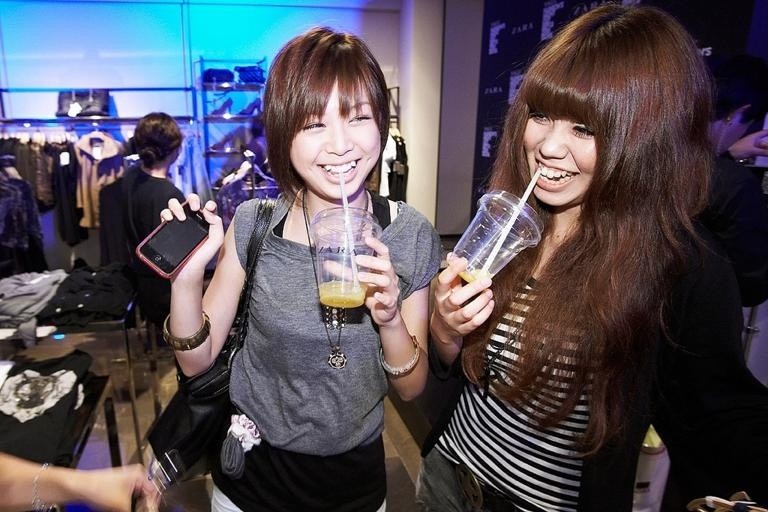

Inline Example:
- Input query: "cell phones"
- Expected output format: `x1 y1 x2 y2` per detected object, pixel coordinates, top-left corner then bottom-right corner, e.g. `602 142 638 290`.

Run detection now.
134 199 210 281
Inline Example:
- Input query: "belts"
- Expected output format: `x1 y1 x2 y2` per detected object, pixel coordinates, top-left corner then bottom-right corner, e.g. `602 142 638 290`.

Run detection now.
451 464 513 511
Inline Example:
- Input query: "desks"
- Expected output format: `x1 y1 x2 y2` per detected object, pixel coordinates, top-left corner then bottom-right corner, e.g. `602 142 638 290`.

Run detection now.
29 374 125 512
0 293 162 463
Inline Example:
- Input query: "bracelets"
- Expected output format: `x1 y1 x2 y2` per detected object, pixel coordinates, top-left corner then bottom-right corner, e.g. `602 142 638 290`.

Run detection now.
380 337 421 375
161 310 213 349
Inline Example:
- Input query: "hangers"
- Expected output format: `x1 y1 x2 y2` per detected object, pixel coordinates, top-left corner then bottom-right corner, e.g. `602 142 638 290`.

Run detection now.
1 122 106 146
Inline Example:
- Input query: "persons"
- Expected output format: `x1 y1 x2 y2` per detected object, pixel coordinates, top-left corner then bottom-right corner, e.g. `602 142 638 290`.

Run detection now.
98 113 187 343
698 126 768 236
414 3 767 512
0 451 159 512
160 20 450 512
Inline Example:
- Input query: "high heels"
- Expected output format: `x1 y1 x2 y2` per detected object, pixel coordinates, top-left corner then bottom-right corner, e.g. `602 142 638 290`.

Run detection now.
210 95 262 115
212 132 234 150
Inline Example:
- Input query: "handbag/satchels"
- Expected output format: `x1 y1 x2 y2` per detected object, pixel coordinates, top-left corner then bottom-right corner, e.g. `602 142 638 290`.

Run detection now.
206 66 264 82
56 90 109 117
143 198 275 485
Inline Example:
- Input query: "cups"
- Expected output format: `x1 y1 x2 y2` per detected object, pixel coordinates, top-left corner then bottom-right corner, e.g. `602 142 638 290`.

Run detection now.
449 188 545 283
308 207 382 308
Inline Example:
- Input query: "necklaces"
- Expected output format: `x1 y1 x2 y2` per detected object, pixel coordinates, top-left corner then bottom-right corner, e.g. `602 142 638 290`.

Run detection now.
300 189 372 374
33 459 58 512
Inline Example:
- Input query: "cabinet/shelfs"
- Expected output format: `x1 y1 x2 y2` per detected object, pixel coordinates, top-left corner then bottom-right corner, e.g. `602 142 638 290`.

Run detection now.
192 56 268 190
